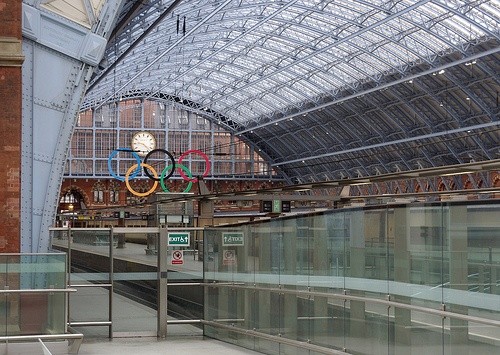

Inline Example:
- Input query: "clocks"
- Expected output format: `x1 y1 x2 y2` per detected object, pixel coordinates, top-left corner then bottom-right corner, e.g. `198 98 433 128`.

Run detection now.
132 131 156 156
